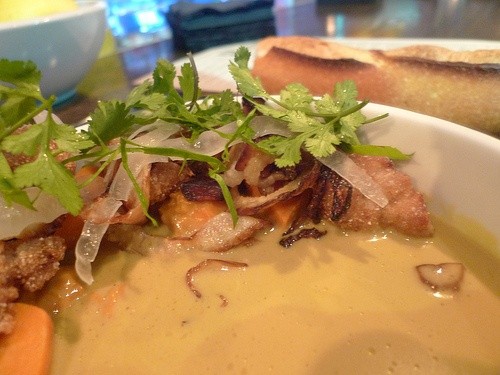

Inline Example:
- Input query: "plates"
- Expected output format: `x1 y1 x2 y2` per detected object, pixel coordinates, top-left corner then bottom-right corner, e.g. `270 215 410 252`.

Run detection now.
131 37 500 133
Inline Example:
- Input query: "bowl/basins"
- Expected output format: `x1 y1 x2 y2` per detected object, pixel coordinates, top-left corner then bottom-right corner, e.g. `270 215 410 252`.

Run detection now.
0 2 108 107
0 94 499 375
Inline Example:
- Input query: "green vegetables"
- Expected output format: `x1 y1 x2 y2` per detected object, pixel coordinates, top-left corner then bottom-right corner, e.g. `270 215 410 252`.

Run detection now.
1 58 415 228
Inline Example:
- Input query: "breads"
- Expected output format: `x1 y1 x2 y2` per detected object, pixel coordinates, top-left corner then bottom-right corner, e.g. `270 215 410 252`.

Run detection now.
249 33 499 135
